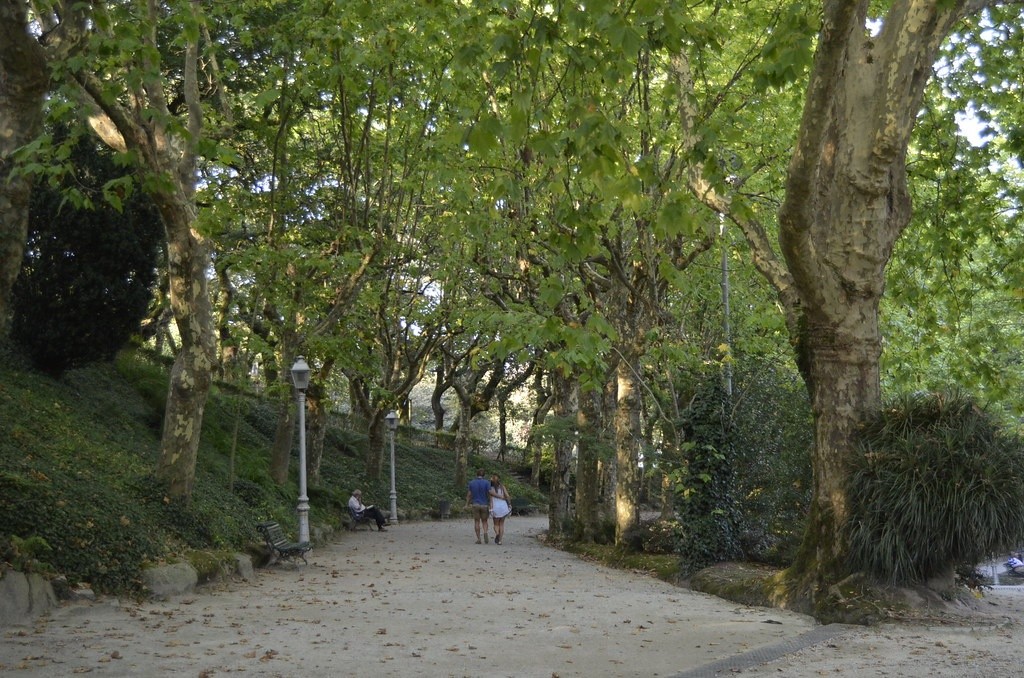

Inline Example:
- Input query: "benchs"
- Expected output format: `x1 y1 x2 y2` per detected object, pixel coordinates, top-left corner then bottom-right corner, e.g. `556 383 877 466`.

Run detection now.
510 498 540 518
256 521 312 571
339 506 374 532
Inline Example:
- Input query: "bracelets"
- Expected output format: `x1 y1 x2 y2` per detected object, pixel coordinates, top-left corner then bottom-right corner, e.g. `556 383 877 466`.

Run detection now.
508 503 511 505
501 496 504 499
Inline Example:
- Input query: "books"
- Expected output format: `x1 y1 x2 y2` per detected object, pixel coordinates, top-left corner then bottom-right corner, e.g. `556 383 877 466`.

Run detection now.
367 504 374 510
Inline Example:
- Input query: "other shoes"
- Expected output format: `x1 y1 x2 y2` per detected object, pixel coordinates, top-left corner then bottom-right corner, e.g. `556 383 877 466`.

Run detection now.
498 542 502 545
495 534 500 543
484 533 489 543
475 541 481 544
383 521 391 527
378 528 385 532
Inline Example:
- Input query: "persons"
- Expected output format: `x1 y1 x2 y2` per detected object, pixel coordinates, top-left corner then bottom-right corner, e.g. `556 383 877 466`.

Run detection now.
349 489 391 532
464 469 512 545
